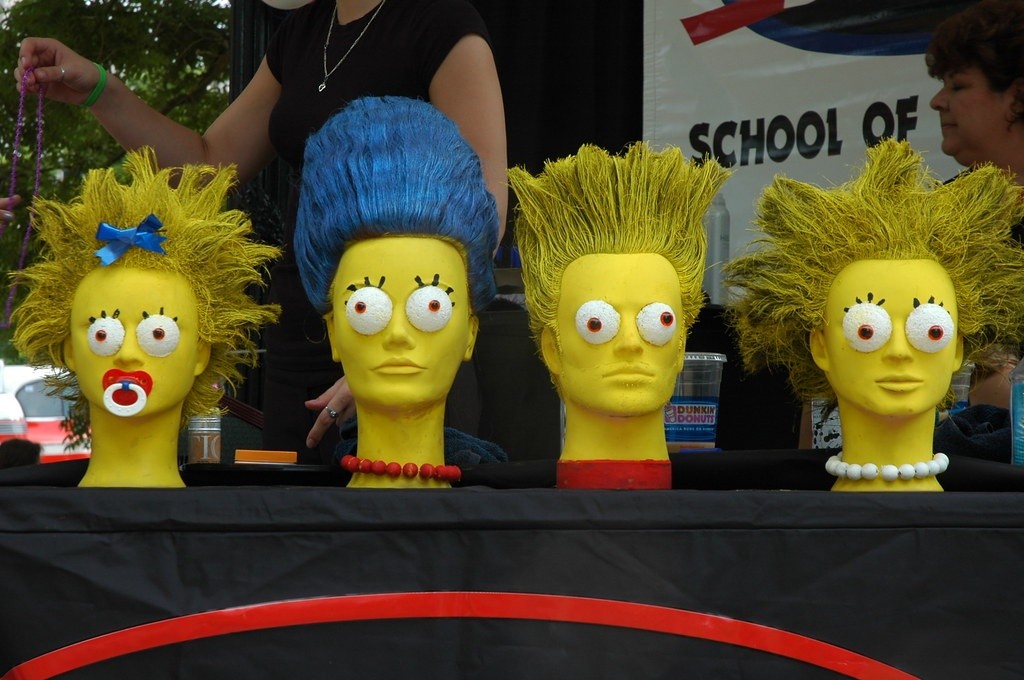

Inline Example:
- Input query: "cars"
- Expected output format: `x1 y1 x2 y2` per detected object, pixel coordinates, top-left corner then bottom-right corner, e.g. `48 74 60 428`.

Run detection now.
0 362 93 490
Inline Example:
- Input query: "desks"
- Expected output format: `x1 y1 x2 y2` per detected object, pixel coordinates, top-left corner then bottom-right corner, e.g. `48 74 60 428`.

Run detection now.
0 485 1024 680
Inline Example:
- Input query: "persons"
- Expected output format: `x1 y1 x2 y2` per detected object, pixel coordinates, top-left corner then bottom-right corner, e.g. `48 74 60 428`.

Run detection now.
6 146 285 488
502 140 734 489
721 135 1024 493
294 96 497 488
927 0 1024 412
0 194 22 235
14 0 508 464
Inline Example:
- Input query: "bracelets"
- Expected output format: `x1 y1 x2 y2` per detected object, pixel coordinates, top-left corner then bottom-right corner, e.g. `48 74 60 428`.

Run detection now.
80 62 107 107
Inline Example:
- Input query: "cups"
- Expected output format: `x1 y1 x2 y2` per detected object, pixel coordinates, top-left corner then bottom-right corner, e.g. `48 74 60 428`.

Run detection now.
663 350 728 451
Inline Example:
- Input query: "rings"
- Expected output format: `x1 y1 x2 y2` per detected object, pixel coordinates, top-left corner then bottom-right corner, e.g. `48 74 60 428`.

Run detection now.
59 65 65 81
324 405 339 417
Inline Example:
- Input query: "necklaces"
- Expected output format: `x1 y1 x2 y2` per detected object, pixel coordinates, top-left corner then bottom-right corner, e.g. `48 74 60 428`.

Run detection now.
341 455 462 482
319 0 386 93
0 66 42 329
826 450 950 481
